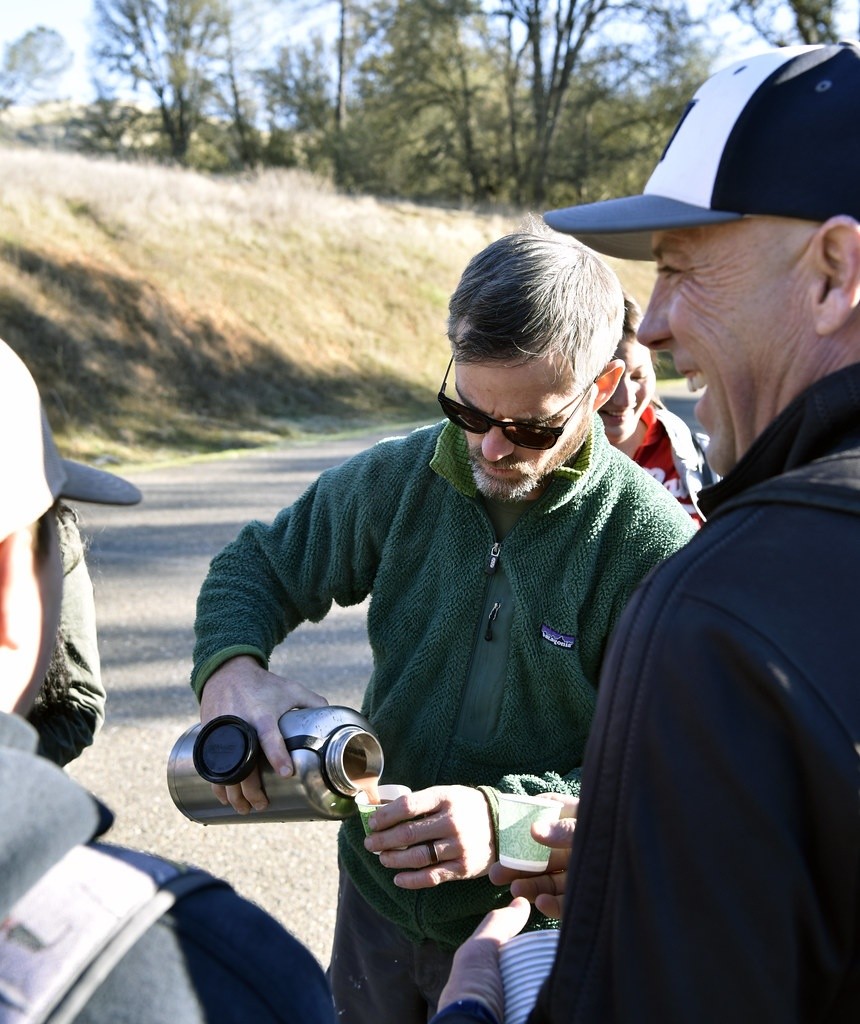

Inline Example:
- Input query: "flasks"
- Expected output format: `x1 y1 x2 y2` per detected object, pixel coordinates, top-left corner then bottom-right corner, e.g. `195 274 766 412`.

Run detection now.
167 706 385 826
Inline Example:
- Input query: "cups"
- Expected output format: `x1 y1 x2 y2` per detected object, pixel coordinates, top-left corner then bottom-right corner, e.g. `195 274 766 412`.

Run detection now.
355 783 411 857
493 791 566 871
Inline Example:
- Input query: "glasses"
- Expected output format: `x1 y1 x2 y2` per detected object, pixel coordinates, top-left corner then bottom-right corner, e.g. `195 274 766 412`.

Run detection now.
437 350 598 450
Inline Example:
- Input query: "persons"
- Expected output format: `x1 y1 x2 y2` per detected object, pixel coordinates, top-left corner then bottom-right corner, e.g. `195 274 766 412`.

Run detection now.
431 44 860 1024
0 334 336 1024
189 233 702 1023
598 288 721 529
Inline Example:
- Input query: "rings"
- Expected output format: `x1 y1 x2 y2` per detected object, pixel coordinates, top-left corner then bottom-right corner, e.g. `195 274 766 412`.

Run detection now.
427 842 437 863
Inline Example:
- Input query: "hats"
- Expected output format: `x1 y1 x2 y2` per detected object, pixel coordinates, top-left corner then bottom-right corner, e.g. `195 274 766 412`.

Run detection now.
542 38 860 262
0 336 142 546
